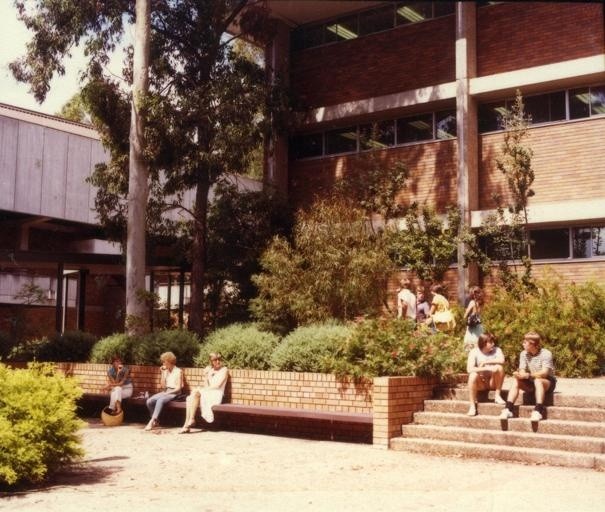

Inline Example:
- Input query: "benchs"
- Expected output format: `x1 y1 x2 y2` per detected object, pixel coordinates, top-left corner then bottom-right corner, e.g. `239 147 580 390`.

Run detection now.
82 394 372 441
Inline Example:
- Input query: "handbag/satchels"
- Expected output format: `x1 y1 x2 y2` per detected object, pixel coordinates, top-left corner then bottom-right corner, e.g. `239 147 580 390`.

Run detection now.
432 310 456 333
466 300 483 328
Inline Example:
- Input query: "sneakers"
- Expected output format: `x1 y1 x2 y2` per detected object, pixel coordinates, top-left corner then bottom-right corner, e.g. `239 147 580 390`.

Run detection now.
530 410 543 421
468 403 476 416
495 395 514 420
144 420 160 431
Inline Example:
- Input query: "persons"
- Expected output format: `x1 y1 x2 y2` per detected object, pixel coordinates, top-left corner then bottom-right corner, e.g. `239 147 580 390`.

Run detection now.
178 352 228 434
106 356 134 415
144 352 184 431
463 286 485 349
429 284 450 336
498 330 556 422
467 331 507 416
397 279 417 323
416 286 432 328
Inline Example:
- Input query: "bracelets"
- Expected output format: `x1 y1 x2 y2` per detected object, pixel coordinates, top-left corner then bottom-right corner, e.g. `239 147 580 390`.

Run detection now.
529 373 531 379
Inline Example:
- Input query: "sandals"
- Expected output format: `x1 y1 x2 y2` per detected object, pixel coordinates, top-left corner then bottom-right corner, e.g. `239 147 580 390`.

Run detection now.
179 416 196 433
